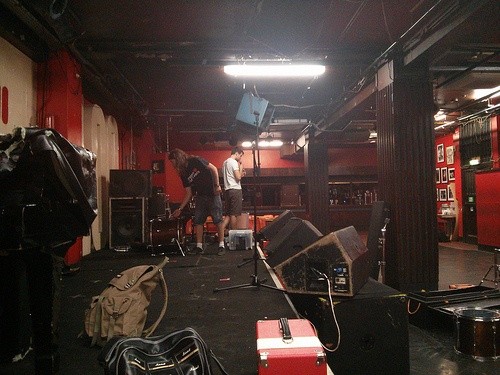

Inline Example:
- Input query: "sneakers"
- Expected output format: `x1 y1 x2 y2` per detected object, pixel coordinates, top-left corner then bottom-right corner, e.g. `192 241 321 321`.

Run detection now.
217 247 225 255
188 247 204 254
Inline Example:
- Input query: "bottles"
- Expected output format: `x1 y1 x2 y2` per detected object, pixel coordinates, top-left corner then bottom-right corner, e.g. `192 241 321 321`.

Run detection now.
343 192 358 208
329 192 343 207
357 190 379 208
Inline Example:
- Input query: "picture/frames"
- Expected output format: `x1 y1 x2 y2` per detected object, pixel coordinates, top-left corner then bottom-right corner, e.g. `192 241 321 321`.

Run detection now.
448 185 455 201
449 168 455 180
436 168 440 183
440 189 446 201
441 167 448 183
437 144 444 162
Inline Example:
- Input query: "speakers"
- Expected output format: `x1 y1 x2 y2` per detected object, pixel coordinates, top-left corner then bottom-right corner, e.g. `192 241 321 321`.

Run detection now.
236 93 275 131
260 209 370 297
109 170 152 198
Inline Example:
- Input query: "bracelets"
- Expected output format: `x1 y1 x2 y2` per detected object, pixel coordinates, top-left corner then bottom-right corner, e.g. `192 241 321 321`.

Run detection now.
178 207 183 212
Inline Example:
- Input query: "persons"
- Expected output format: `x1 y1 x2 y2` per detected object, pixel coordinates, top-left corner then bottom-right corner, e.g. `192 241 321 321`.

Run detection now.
215 146 247 248
168 148 225 256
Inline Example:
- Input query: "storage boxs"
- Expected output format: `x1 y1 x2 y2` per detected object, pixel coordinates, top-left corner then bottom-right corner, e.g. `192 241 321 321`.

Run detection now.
229 230 253 250
256 318 327 375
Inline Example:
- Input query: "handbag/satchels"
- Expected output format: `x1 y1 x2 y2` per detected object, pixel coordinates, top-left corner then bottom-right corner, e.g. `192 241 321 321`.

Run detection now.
106 326 228 375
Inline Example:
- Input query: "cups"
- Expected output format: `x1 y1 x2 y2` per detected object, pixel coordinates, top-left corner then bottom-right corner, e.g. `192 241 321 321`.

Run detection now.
165 208 171 220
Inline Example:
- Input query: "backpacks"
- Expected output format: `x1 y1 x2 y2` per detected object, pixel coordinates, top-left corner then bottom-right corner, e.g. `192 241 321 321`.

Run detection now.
84 256 169 346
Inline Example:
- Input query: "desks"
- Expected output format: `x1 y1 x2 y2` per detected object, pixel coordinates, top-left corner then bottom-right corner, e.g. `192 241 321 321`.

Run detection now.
438 214 456 241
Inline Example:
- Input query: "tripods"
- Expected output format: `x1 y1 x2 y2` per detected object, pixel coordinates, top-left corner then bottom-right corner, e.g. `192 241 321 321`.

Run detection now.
212 113 289 296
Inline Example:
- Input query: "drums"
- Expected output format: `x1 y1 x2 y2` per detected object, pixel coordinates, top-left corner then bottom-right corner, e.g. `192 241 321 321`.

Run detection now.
454 307 500 362
150 218 182 255
148 193 169 218
